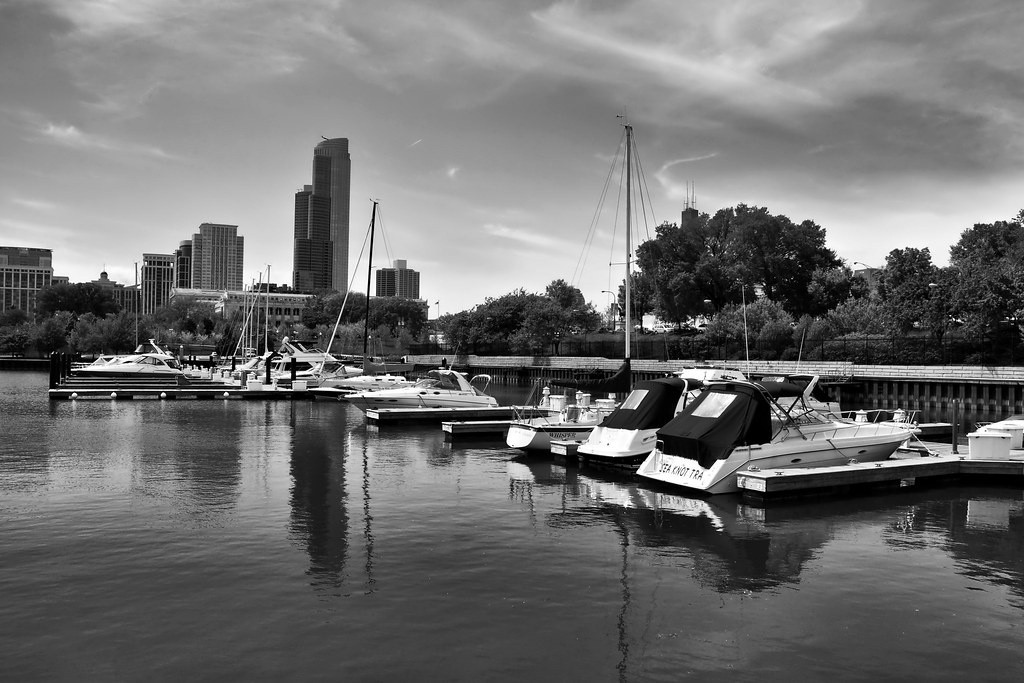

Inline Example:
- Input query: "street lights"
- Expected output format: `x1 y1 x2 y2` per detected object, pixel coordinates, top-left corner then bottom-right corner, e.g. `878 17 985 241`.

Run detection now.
929 283 946 366
854 262 875 277
368 334 372 358
704 296 712 359
602 291 616 334
435 300 440 318
319 331 322 349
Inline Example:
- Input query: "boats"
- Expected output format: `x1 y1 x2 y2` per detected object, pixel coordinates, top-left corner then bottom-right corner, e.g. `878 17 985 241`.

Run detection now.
506 391 626 450
576 377 707 463
228 337 364 389
635 372 922 495
343 367 500 413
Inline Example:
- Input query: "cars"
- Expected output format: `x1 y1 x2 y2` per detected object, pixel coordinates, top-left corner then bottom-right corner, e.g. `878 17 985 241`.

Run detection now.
673 324 698 334
242 348 256 357
650 324 675 334
211 352 217 356
697 323 709 333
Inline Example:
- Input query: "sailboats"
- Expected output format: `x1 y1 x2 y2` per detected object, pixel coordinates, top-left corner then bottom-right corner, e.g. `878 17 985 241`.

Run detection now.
308 202 442 397
71 261 192 377
506 124 843 451
221 264 282 379
